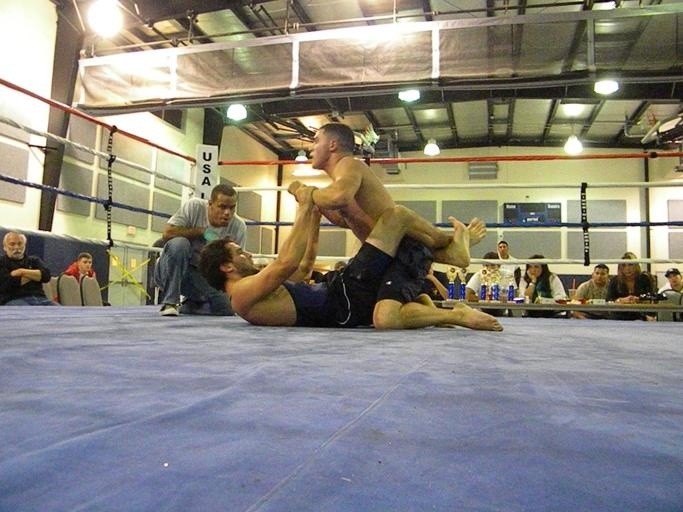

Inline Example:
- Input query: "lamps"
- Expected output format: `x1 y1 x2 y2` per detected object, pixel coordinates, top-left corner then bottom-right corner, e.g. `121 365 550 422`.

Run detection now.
225 103 248 122
562 119 585 157
593 80 618 97
397 89 420 103
422 121 443 157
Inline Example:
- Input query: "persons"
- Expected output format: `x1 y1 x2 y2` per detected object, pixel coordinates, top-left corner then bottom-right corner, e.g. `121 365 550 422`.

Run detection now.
448 240 682 321
0 231 62 307
196 184 487 328
420 266 448 301
286 122 504 332
153 183 246 317
64 251 95 283
334 261 346 270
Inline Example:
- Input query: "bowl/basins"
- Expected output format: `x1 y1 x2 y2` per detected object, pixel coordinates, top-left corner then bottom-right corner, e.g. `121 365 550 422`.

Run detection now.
514 298 524 304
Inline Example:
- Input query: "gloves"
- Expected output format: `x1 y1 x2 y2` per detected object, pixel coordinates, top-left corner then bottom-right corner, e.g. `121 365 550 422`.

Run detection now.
204 229 220 242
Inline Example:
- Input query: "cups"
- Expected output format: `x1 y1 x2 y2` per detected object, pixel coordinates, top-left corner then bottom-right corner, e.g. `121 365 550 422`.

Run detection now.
568 288 578 302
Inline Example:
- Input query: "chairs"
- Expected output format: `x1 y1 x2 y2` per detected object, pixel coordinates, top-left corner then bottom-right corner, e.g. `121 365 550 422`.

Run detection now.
43 275 103 306
653 291 683 322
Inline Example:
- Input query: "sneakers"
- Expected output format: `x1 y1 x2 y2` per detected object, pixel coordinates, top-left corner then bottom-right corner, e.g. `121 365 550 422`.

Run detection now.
160 303 180 316
181 294 206 314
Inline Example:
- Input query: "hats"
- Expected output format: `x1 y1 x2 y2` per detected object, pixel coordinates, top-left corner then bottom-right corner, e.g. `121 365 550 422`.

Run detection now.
665 268 680 277
